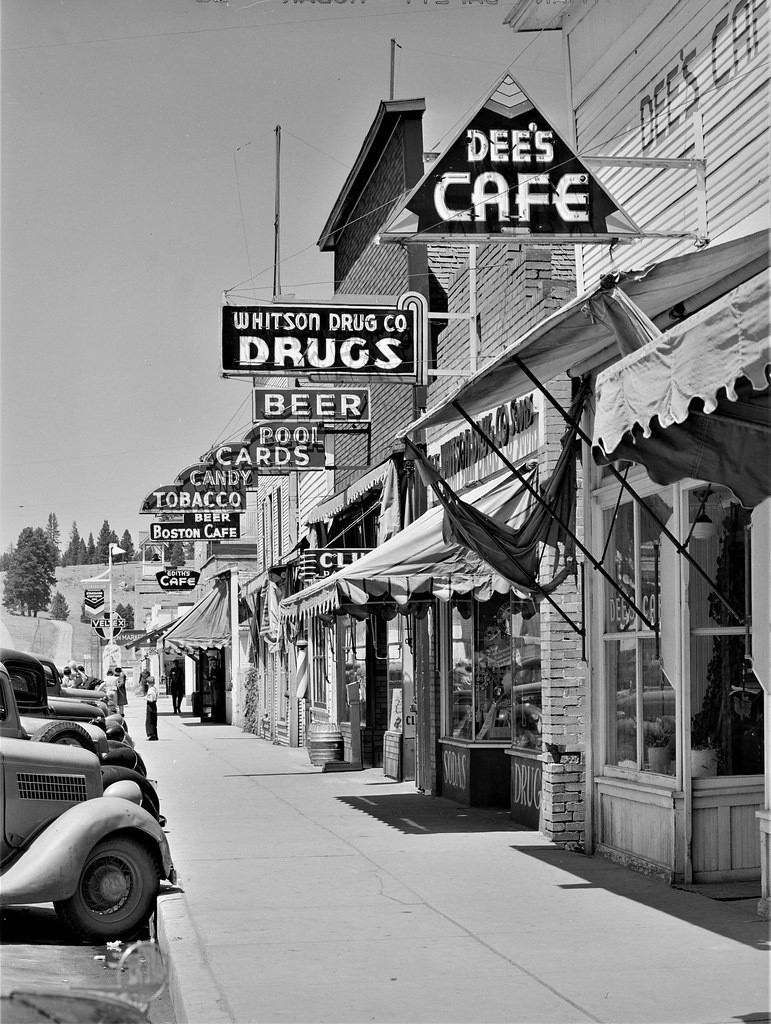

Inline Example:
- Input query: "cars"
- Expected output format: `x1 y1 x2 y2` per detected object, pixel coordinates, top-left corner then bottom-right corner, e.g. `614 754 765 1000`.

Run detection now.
444 650 675 755
0 647 168 825
0 660 178 949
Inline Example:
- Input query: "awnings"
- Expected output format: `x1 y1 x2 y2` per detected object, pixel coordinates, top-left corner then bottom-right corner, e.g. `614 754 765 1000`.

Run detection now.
124 616 181 650
280 460 537 622
592 266 771 509
156 581 233 656
308 461 391 524
395 228 771 635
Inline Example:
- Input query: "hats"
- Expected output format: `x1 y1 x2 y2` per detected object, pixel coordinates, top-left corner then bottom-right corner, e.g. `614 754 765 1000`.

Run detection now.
115 668 122 672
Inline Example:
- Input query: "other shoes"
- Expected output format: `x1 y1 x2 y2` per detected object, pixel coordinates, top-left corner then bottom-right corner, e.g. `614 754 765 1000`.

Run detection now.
174 709 177 714
178 708 181 713
147 736 158 740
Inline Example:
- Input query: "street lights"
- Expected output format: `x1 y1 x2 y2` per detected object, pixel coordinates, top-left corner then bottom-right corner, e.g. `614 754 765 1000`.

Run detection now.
109 543 127 644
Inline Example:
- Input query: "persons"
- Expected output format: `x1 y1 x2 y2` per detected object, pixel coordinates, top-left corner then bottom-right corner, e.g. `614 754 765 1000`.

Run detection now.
139 668 150 696
143 676 159 741
105 668 127 717
61 662 87 688
169 659 186 715
204 658 220 682
